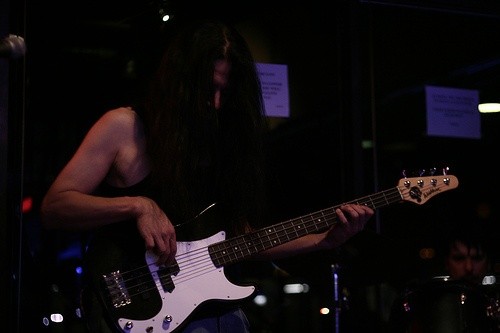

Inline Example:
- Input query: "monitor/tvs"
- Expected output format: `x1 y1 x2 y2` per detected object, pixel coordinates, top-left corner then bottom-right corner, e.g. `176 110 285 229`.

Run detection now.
425 85 482 139
254 62 290 117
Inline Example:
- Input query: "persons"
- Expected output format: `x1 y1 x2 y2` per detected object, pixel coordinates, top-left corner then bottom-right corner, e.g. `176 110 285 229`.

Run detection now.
41 23 374 333
388 240 500 333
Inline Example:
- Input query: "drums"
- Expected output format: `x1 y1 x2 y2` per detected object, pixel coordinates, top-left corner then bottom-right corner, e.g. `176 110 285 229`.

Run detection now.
390 275 500 333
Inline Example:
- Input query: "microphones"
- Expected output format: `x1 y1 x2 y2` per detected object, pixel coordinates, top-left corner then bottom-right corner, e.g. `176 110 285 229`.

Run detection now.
330 262 342 308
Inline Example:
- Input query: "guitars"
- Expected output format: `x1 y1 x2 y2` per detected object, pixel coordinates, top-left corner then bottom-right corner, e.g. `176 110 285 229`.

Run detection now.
83 166 459 333
